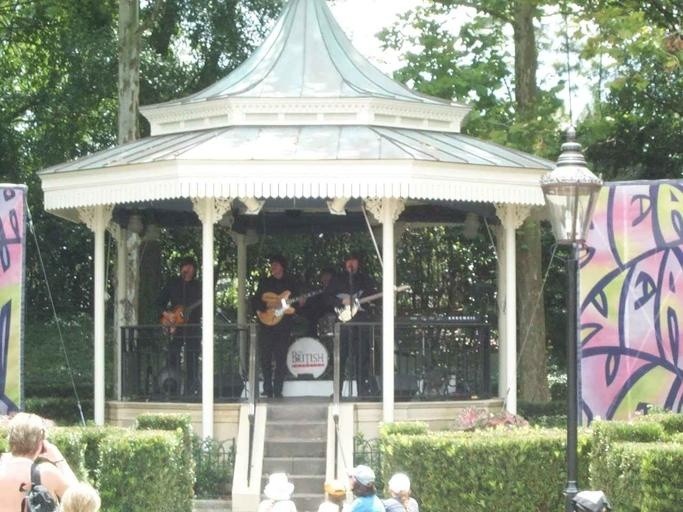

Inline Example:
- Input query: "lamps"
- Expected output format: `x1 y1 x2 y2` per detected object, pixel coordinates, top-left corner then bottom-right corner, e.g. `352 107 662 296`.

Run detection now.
126 205 143 234
461 211 480 241
285 182 302 218
238 196 265 216
326 195 351 216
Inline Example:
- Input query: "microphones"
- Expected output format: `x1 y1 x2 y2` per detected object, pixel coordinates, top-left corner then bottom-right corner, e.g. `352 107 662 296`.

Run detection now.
182 271 187 281
348 266 352 277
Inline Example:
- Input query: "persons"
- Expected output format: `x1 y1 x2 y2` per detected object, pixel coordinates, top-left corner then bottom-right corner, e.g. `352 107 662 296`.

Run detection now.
382 473 418 512
51 481 101 512
345 465 385 511
571 489 612 511
153 256 201 400
320 267 336 288
258 471 297 511
252 254 308 398
324 252 379 396
317 478 349 512
0 412 77 512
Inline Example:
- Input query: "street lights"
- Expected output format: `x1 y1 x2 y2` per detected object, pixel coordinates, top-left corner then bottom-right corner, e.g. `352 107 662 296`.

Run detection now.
539 124 603 512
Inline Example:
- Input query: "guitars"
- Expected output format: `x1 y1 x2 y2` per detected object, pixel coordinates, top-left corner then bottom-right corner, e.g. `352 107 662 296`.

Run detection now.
256 287 327 326
334 283 411 322
161 299 202 337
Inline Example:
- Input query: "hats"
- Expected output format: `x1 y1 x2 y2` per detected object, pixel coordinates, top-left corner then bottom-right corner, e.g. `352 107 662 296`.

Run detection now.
264 471 297 500
345 464 375 487
387 471 411 495
321 479 347 497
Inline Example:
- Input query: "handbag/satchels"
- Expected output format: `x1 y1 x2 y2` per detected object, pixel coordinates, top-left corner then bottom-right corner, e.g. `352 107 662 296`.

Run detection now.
19 456 58 512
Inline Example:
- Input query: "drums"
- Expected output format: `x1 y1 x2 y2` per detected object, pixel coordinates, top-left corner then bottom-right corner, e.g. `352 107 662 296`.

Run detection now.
316 313 336 338
286 335 330 380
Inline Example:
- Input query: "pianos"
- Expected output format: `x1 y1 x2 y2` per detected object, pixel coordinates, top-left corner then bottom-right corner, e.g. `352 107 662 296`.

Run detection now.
409 315 482 322
